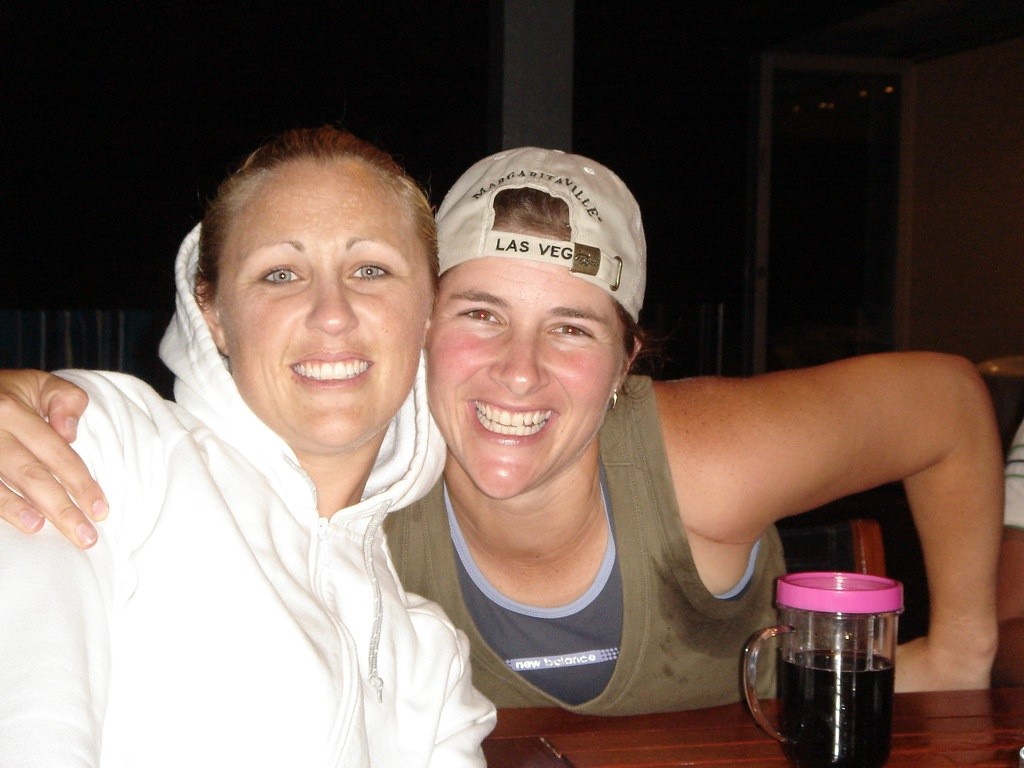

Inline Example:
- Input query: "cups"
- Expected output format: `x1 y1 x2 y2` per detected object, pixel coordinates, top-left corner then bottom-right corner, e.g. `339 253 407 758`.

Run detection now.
741 571 904 768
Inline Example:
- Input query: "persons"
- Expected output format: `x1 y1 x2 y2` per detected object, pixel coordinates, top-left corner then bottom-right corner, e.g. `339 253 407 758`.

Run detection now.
0 148 1004 692
0 129 497 768
994 419 1024 686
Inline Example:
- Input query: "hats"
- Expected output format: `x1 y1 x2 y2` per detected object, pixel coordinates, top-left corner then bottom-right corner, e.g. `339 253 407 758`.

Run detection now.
434 146 647 324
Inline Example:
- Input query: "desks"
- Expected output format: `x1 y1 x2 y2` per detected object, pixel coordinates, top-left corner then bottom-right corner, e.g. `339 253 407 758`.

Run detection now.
482 683 1024 768
